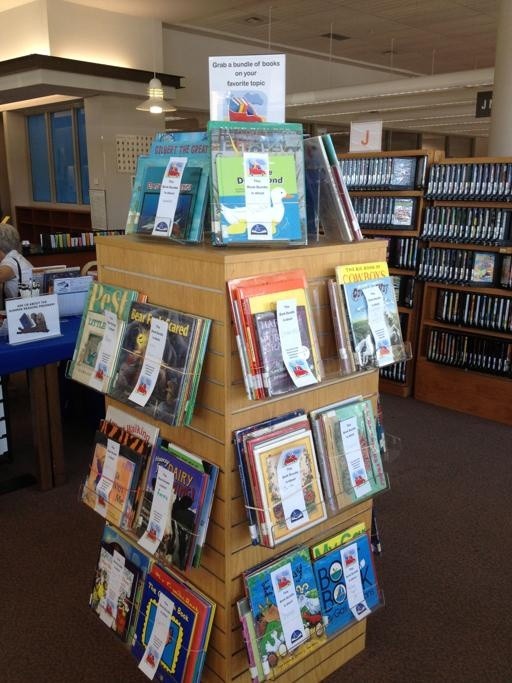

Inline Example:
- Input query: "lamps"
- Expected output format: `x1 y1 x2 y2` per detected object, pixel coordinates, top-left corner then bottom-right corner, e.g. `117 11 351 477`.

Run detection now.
133 0 179 114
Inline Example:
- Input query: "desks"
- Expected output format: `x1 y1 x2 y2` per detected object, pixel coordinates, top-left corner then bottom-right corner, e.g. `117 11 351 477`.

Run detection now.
1 314 87 493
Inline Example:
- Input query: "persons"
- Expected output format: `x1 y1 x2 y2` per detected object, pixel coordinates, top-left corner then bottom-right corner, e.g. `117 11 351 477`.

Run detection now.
1 222 35 298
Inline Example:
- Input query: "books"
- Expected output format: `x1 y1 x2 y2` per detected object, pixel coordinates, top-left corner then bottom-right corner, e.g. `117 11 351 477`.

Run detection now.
68 280 212 427
226 269 326 400
81 405 219 573
328 159 416 382
232 393 392 555
237 522 385 682
30 264 65 318
65 231 123 317
39 231 65 249
88 524 216 682
125 54 364 248
410 156 511 385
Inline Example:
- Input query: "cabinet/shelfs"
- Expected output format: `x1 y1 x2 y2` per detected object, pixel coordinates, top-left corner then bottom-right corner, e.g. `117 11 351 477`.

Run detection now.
15 205 93 244
93 227 394 681
